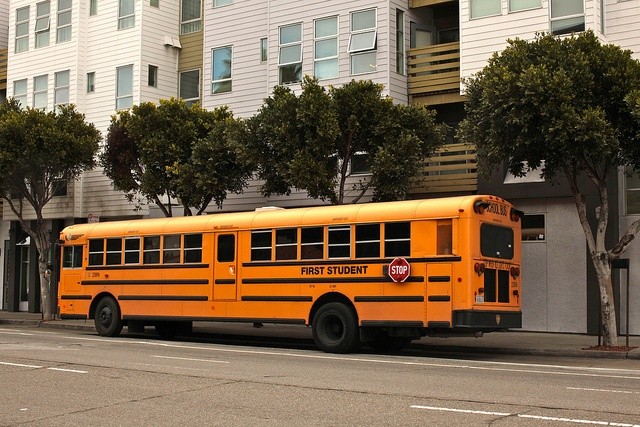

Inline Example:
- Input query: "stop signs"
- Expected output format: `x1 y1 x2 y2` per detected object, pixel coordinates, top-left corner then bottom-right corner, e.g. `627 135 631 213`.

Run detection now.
388 259 410 282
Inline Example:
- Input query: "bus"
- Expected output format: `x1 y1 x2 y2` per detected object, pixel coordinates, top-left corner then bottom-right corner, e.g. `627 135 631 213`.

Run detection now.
56 195 524 354
